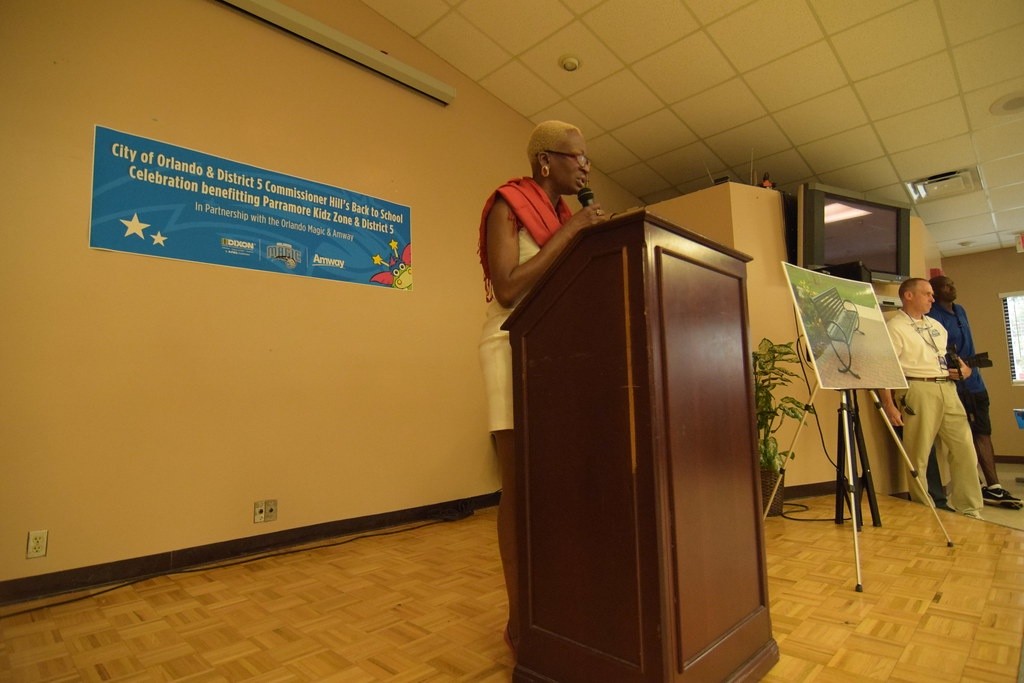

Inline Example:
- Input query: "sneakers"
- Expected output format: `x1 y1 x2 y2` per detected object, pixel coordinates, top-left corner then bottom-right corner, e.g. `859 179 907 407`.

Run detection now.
983 488 1023 510
956 510 985 520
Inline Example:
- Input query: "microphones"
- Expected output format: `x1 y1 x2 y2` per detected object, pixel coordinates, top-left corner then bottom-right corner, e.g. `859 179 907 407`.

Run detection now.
577 188 594 208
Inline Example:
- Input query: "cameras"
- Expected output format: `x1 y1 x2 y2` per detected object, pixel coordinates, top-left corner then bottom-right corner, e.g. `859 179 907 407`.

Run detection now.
944 352 992 368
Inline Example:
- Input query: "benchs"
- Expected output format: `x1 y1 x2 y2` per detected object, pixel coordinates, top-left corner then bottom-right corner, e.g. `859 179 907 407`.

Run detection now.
809 285 865 380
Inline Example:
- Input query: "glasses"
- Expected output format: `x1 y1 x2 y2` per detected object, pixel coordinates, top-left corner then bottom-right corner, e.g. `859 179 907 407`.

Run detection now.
536 150 592 167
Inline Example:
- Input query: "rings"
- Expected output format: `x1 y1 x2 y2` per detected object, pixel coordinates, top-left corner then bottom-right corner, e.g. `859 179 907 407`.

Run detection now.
597 209 601 216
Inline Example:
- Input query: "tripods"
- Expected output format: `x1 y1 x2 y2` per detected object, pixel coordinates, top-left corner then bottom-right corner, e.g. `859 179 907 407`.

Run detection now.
834 389 882 529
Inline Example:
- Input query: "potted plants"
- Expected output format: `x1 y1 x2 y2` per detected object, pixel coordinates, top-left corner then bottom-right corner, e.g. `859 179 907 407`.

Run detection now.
752 338 816 516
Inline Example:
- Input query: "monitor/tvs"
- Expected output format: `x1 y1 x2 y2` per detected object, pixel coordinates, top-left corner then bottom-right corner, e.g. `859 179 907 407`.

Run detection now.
798 182 911 284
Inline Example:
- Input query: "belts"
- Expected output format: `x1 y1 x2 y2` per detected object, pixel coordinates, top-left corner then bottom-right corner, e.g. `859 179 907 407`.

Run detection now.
906 376 949 382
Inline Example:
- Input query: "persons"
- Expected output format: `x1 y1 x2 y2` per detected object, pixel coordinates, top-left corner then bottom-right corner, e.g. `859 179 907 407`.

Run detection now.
480 120 607 659
878 276 1023 519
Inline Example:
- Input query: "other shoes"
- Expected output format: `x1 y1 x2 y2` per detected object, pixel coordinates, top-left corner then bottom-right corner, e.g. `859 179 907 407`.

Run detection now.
503 623 518 660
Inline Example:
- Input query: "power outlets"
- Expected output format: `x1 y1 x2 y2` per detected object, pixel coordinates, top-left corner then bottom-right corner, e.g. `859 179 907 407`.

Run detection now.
26 530 48 559
